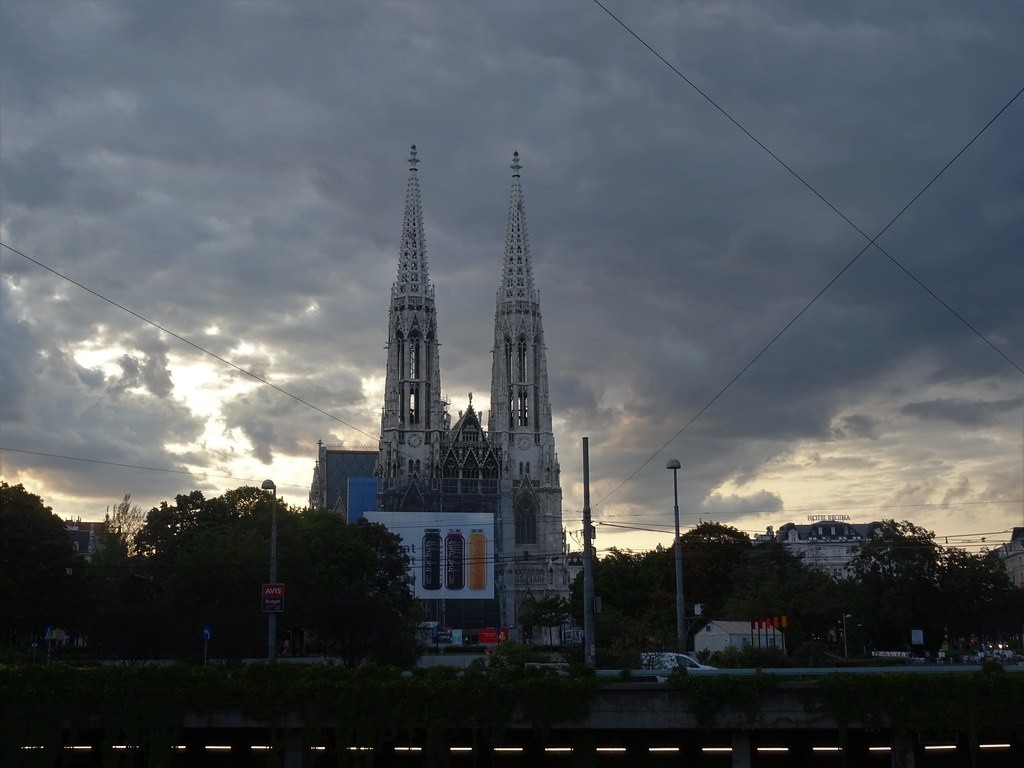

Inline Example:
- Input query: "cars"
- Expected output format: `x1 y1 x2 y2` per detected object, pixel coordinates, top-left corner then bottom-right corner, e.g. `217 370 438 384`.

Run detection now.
639 652 718 685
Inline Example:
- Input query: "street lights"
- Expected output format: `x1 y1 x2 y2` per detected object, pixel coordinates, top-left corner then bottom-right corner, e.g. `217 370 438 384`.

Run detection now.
842 613 852 659
261 480 276 659
287 630 293 658
665 458 688 653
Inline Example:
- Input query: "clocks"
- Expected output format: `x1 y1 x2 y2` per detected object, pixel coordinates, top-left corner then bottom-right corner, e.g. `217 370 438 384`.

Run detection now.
517 436 532 450
407 433 422 448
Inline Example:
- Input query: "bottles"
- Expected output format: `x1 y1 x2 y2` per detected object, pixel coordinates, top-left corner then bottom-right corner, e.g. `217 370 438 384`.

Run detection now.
444 528 465 590
422 528 445 590
468 528 487 592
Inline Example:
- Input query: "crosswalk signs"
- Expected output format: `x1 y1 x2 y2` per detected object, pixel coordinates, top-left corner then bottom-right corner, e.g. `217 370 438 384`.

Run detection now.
43 627 54 640
